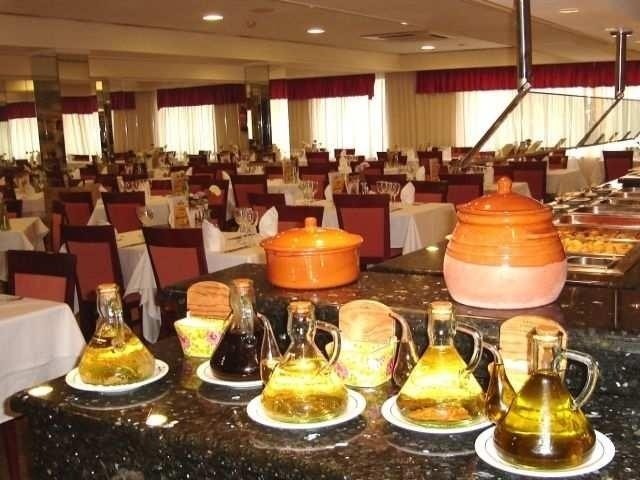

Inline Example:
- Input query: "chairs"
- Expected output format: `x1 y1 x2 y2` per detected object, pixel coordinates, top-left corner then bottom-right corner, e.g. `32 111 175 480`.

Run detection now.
0 146 570 343
602 150 633 182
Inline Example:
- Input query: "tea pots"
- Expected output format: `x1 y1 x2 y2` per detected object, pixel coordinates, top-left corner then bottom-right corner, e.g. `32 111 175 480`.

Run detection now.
80 283 156 383
255 301 349 423
483 323 600 468
387 300 485 426
209 279 283 383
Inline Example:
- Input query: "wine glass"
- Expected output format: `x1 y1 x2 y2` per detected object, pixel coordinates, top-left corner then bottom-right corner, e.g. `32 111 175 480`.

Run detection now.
299 180 319 202
357 180 400 211
234 207 258 245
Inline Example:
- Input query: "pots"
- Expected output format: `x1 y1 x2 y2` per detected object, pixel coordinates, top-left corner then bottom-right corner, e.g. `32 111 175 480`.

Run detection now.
443 178 568 310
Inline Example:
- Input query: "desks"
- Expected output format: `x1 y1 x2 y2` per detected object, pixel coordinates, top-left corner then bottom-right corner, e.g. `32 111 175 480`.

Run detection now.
0 293 88 426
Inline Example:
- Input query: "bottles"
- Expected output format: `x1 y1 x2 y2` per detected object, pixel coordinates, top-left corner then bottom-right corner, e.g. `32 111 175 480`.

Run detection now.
2 215 11 231
196 208 209 224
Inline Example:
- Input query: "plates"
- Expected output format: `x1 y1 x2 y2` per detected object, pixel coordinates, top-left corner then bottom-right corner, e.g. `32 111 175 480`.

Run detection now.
379 394 497 434
197 359 265 391
69 384 172 411
246 388 367 431
545 188 614 210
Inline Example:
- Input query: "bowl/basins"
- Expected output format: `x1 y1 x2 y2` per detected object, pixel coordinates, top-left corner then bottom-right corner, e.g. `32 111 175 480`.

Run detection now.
259 218 364 289
